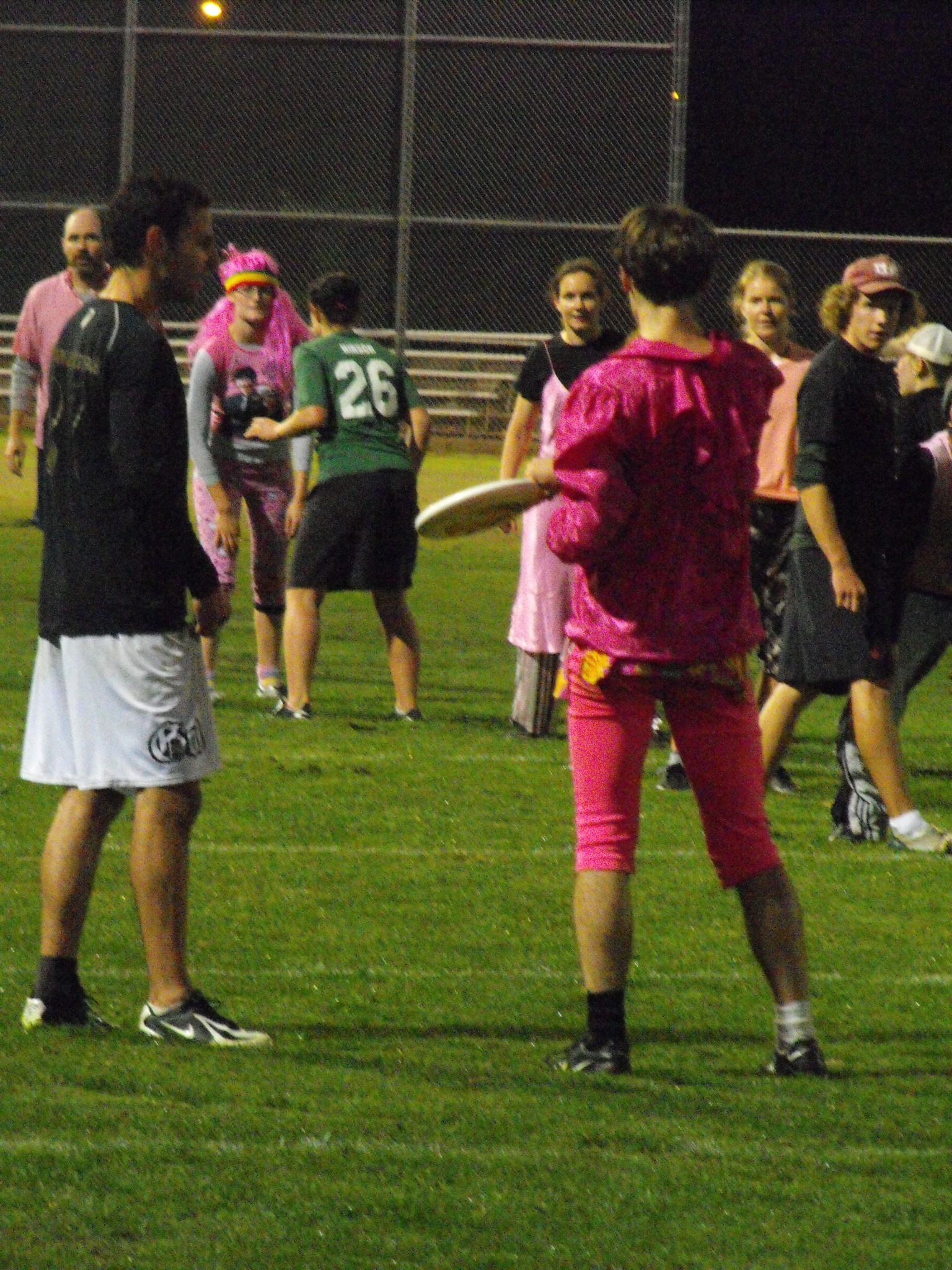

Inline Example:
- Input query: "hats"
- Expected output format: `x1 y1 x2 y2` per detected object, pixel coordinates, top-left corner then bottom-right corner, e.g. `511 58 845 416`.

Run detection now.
843 253 917 298
889 320 951 367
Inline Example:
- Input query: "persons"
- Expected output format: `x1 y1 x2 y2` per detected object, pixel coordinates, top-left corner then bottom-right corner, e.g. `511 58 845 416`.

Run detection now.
184 248 319 712
527 204 833 1084
20 167 274 1055
243 275 428 726
6 203 116 519
493 256 625 741
658 254 952 859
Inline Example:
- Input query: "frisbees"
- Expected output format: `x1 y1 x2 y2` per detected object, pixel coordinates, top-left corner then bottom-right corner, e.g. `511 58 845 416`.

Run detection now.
415 477 545 544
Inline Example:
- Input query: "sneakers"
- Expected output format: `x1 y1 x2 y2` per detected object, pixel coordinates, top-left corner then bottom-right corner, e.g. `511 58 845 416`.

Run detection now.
657 763 691 789
757 1037 829 1077
266 699 312 720
20 987 122 1032
137 988 275 1048
254 684 289 700
541 1038 636 1075
383 706 425 722
887 825 952 853
767 765 805 796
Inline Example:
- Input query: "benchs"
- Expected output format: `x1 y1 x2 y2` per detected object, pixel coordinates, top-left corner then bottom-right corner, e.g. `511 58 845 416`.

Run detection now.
0 313 554 450
